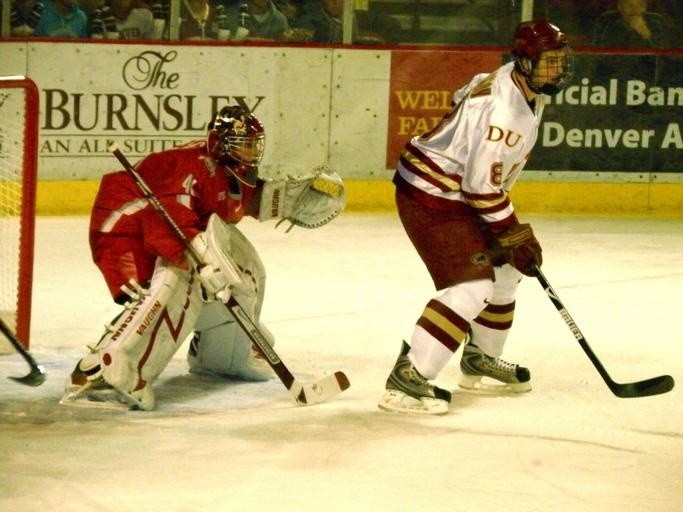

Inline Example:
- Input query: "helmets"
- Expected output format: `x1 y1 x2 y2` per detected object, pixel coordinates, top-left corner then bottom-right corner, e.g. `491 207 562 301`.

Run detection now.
508 21 573 95
207 109 265 188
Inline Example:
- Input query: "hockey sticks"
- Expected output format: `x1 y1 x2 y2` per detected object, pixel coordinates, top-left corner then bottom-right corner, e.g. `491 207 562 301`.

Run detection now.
532 265 674 398
108 142 350 404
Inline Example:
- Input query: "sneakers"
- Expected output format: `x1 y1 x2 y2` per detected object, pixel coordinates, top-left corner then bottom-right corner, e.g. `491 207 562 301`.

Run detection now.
386 342 451 400
461 329 530 384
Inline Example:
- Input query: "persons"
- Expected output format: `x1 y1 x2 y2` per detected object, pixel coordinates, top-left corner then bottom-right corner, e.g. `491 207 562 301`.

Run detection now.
385 19 574 403
592 0 670 49
64 105 346 410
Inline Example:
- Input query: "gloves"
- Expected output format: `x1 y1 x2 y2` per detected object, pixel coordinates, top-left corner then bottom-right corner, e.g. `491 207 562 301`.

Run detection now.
497 225 544 277
